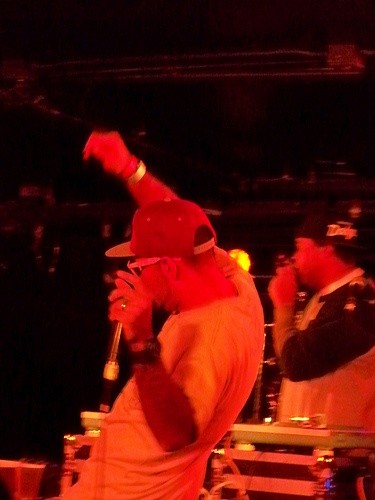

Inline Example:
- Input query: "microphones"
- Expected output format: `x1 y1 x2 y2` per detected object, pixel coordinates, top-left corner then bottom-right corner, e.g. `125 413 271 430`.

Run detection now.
102 320 123 382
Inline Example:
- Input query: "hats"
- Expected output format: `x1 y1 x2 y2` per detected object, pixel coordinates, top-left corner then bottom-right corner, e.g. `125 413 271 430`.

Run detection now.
104 199 217 258
285 199 362 247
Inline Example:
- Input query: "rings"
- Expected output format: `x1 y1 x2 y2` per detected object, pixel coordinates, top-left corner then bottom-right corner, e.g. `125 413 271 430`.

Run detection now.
121 299 129 310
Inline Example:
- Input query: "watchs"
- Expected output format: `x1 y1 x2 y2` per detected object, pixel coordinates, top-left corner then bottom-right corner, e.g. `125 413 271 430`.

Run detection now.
129 333 162 365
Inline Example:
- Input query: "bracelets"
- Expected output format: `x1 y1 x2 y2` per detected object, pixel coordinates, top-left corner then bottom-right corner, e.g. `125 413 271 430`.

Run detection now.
120 159 147 188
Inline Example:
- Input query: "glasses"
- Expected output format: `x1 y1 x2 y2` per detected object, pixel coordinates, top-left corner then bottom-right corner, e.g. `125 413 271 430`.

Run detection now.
127 257 180 277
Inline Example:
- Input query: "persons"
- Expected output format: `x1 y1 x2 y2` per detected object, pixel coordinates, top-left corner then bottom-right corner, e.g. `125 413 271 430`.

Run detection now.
60 131 266 499
267 207 375 431
